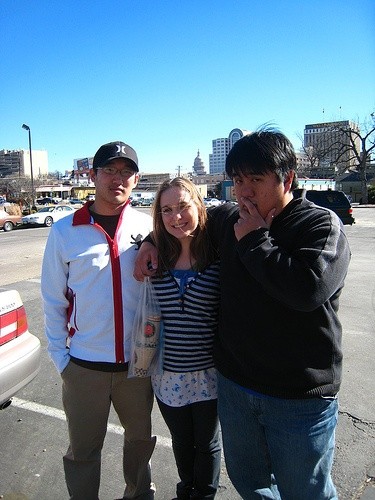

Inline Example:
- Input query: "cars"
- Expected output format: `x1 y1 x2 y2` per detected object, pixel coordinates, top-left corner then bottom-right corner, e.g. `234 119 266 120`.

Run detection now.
20 205 76 227
202 199 221 208
35 195 94 206
0 287 42 410
0 210 16 232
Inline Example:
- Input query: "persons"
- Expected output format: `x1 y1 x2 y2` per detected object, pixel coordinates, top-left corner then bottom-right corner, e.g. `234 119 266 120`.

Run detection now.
40 142 160 500
134 128 351 500
133 177 222 500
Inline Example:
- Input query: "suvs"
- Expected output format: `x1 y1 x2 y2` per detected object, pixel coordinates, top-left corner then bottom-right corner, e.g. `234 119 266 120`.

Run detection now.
306 188 355 227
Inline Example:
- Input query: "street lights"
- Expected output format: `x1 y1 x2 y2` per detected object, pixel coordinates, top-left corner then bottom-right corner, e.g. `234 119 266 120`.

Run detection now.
22 124 35 205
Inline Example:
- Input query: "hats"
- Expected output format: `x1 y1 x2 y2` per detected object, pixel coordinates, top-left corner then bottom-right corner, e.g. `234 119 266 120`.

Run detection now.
93 141 139 173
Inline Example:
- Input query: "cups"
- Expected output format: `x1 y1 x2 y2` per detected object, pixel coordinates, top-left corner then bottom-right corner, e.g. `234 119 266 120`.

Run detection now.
129 313 162 378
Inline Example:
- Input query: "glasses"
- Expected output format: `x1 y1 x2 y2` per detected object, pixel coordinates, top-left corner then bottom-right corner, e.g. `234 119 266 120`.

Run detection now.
96 167 137 178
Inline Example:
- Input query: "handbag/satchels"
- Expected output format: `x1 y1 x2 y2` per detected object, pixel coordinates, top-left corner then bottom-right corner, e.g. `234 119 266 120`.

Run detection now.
126 276 166 379
